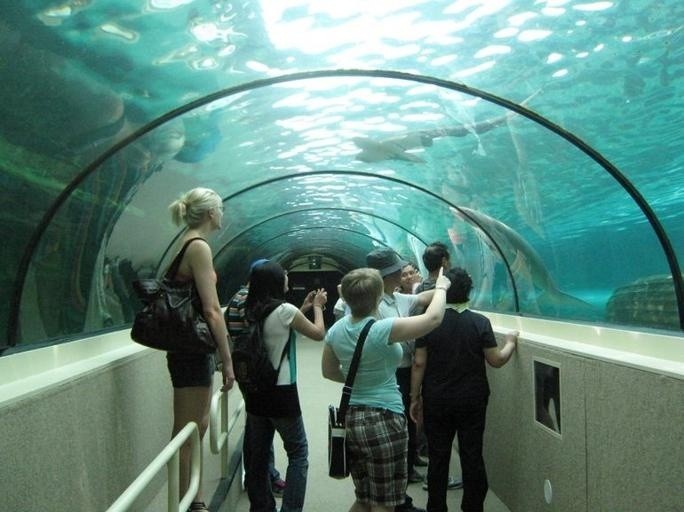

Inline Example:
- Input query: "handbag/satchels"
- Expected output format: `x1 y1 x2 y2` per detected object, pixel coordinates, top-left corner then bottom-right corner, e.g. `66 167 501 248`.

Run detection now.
326 403 351 480
131 236 223 356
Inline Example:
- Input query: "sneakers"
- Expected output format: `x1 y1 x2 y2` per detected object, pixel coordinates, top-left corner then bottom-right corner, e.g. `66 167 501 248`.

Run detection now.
414 455 429 467
272 479 287 497
395 493 427 512
422 475 464 493
408 468 424 484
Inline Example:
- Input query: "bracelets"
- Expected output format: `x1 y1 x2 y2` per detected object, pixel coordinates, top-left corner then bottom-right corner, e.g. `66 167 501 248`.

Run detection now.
410 394 420 401
312 301 326 311
435 286 449 293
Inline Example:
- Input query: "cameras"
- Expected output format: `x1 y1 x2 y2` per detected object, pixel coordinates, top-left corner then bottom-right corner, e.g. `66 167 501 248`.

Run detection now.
311 290 327 313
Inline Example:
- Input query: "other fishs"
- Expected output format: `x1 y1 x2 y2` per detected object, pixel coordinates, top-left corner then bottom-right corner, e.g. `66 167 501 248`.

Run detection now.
406 86 544 139
350 133 430 165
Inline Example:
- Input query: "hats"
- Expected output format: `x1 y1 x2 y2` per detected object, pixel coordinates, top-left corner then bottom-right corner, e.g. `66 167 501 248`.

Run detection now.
366 248 409 278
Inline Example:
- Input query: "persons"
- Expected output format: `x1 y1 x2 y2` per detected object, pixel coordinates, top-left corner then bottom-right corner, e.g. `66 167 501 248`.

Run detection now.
157 186 233 511
332 240 463 491
223 258 287 497
240 258 327 511
321 267 451 512
408 268 519 510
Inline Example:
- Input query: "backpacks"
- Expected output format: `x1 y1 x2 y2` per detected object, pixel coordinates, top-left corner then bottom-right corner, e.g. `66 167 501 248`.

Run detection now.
230 299 292 396
